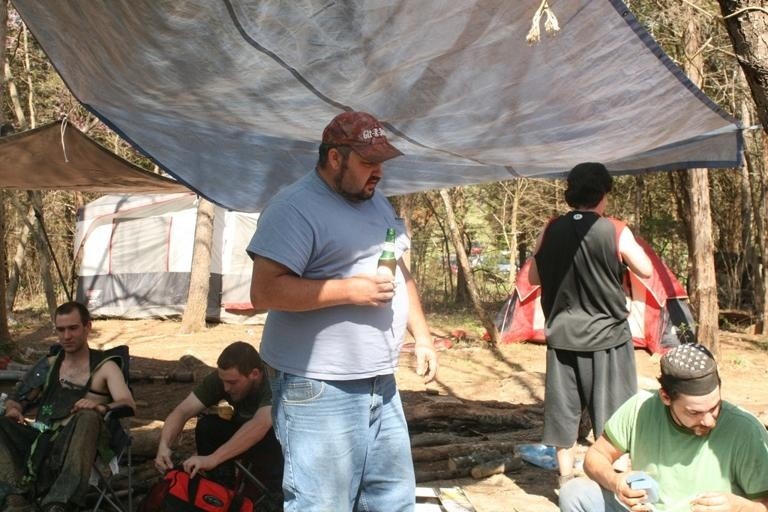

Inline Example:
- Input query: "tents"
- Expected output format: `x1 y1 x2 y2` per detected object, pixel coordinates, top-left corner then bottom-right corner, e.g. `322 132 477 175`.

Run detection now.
71 191 270 324
496 233 700 357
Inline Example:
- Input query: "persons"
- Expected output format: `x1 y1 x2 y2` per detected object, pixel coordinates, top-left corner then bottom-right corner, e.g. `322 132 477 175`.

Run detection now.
514 162 653 493
245 112 438 512
0 301 135 512
559 343 768 511
154 342 284 512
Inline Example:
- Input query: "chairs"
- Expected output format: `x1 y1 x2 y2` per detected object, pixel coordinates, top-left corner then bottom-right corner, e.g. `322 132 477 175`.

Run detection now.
50 343 132 512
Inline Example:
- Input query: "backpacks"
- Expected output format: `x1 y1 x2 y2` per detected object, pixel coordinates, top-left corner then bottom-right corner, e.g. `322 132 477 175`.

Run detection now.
137 469 252 511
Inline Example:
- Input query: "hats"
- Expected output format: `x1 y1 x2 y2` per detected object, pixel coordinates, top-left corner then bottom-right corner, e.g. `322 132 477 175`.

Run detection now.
321 113 405 163
660 344 719 397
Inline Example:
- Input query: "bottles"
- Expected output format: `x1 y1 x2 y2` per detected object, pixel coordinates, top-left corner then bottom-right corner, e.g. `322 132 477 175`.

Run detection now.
371 229 396 303
